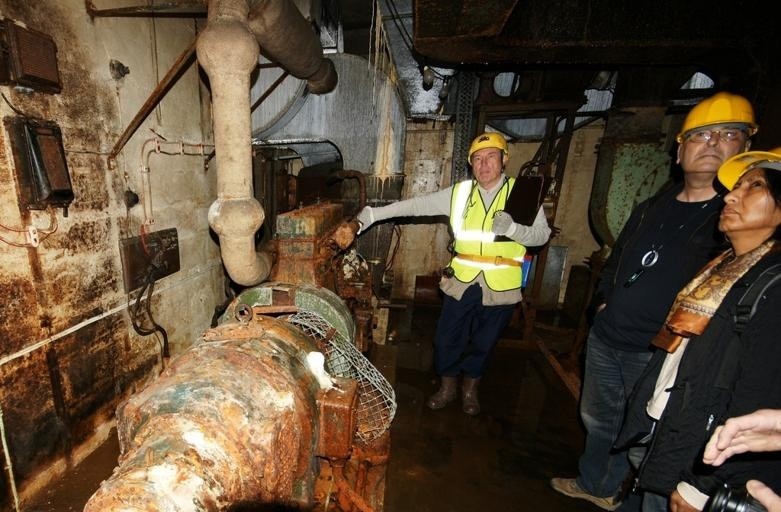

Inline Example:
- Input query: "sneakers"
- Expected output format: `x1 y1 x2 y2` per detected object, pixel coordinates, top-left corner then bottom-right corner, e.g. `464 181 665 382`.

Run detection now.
553 476 622 511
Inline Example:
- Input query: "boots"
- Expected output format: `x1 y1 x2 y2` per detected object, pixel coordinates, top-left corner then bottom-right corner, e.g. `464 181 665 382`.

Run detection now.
427 374 461 410
460 376 481 416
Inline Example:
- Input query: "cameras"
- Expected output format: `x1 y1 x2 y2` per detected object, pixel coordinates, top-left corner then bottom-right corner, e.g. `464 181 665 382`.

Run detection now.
699 481 770 512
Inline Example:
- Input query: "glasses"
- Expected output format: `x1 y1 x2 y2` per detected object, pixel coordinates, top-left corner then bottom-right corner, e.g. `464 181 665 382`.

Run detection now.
681 129 743 145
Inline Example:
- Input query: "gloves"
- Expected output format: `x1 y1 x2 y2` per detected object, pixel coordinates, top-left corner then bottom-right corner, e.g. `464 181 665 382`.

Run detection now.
493 211 518 239
357 206 373 233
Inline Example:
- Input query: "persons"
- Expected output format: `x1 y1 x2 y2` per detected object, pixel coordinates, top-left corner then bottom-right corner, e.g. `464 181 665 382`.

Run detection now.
701 409 781 511
550 92 759 511
610 147 781 512
352 132 551 417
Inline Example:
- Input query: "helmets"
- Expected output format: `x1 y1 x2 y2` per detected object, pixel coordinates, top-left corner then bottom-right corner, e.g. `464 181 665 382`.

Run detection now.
468 133 510 164
677 91 758 148
719 148 781 190
